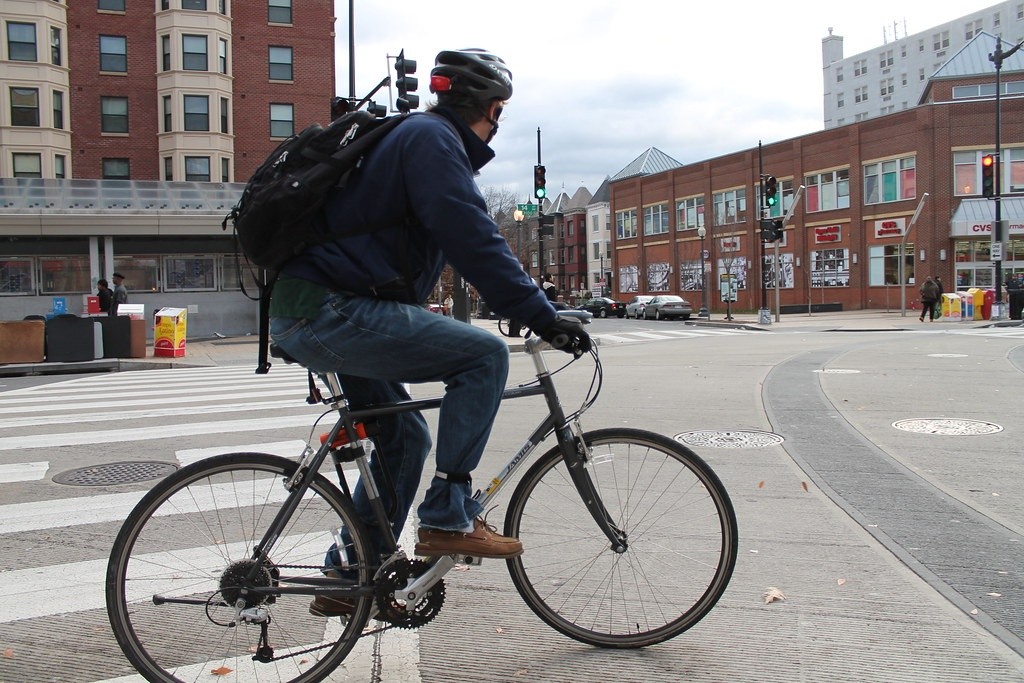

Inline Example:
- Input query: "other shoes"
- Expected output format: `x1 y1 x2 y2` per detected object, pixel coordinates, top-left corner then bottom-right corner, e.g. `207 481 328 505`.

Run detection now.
919 317 924 322
930 319 933 322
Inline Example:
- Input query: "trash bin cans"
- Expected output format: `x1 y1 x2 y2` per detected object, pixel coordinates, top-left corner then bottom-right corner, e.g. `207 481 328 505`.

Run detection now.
1006 289 1024 320
477 299 490 319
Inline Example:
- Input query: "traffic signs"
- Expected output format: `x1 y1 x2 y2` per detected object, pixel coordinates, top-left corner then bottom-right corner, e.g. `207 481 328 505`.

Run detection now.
990 242 1003 261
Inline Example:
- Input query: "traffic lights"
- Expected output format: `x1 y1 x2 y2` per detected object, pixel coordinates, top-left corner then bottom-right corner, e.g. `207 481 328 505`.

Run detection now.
367 101 387 118
981 155 994 198
330 97 347 122
765 175 777 206
394 48 419 113
534 165 546 199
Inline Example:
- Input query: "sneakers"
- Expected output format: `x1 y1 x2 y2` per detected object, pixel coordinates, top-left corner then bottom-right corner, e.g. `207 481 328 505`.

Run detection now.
414 514 524 558
308 568 407 622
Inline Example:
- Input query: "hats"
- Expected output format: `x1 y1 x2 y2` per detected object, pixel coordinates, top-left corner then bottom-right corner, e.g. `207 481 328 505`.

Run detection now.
112 272 126 280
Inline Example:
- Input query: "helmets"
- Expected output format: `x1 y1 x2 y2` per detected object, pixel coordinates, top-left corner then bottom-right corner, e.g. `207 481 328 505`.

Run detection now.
429 48 513 105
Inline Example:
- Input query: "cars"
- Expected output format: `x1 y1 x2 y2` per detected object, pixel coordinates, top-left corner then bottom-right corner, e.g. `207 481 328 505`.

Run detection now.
573 297 627 318
642 294 693 320
625 295 656 319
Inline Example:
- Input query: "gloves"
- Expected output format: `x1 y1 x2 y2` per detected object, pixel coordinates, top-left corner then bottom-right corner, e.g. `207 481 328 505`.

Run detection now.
542 314 592 359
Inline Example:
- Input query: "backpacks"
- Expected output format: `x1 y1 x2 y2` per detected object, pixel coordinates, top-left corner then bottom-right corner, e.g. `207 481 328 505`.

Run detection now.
922 282 936 299
222 111 466 267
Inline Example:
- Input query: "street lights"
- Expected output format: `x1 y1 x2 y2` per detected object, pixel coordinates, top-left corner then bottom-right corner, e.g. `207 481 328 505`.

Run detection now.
697 225 711 318
599 248 605 297
513 207 524 264
774 184 806 322
900 192 930 317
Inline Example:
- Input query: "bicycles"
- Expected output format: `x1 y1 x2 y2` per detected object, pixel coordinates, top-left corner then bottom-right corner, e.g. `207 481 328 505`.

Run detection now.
103 320 741 683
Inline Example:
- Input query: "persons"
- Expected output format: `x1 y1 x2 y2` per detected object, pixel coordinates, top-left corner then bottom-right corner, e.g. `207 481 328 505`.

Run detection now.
919 276 940 322
543 273 556 302
1006 275 1020 289
97 279 112 314
269 47 591 618
444 294 453 318
108 273 127 316
933 276 944 293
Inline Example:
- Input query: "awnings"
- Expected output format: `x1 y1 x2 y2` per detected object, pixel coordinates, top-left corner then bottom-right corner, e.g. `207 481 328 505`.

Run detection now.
951 197 1024 235
